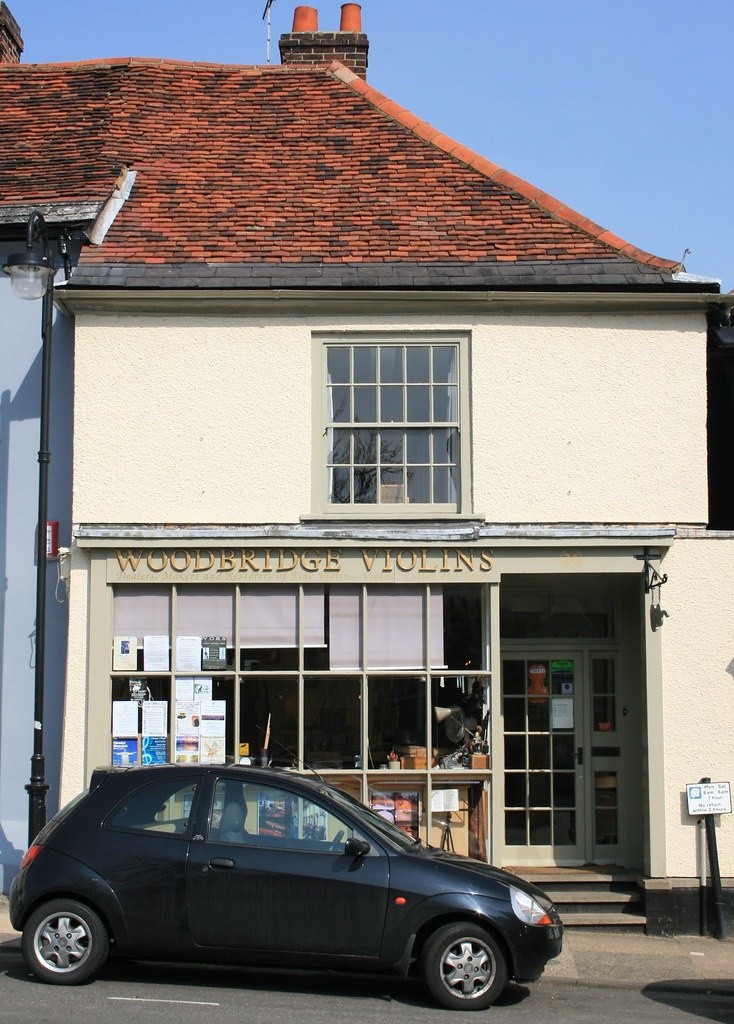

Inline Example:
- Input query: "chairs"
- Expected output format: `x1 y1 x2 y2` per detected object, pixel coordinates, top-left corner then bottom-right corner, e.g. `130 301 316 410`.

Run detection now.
218 797 257 846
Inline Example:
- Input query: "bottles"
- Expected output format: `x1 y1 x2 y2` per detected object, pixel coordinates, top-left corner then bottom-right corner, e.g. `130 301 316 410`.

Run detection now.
389 747 398 762
379 764 388 769
260 749 267 766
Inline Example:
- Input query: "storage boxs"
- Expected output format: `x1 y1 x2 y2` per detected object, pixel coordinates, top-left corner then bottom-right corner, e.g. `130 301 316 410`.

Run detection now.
468 754 489 769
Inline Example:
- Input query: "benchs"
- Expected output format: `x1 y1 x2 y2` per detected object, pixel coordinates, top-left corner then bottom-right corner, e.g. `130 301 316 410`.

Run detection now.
128 817 189 834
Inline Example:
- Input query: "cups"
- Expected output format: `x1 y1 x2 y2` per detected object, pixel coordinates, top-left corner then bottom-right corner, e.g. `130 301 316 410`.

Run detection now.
389 761 400 769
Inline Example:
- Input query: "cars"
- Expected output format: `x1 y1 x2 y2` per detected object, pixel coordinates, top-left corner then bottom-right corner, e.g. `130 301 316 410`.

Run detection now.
6 764 568 1014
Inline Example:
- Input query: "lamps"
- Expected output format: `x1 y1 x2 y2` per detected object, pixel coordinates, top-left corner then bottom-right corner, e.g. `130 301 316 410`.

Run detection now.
434 705 465 742
